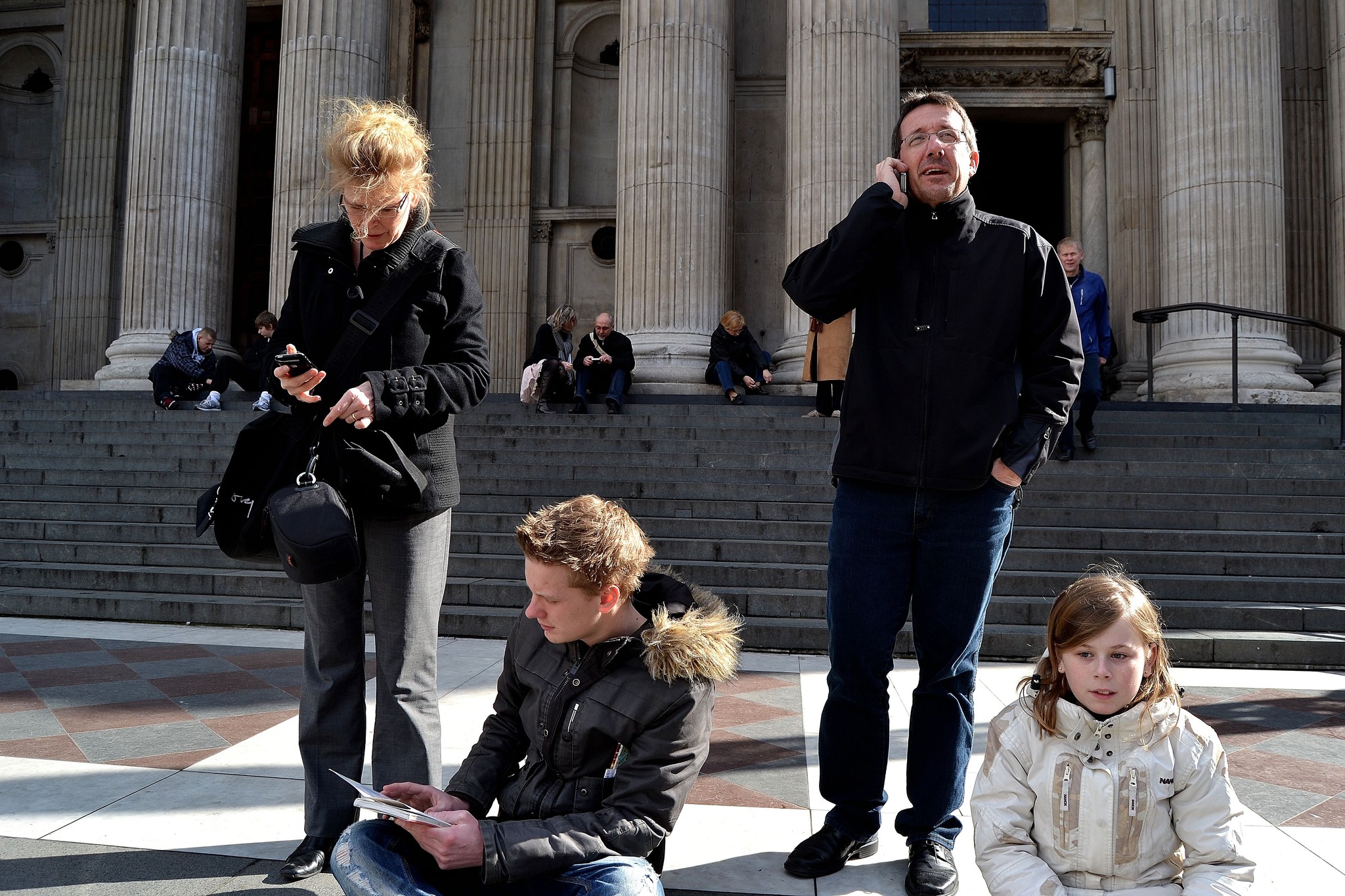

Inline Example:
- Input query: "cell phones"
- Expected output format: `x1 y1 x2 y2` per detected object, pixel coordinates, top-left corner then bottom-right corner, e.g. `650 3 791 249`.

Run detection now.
899 169 910 193
275 352 319 383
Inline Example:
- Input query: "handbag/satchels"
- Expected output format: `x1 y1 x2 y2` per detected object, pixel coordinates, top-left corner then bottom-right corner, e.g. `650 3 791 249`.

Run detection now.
212 404 339 565
259 471 362 585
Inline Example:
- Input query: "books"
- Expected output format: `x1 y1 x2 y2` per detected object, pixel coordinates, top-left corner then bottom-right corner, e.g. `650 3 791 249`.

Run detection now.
329 768 455 831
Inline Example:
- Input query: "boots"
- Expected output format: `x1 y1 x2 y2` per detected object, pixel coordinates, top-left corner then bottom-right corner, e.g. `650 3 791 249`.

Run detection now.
535 371 557 414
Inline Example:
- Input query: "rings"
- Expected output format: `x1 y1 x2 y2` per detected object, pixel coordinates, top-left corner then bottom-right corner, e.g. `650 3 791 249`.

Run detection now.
352 413 359 421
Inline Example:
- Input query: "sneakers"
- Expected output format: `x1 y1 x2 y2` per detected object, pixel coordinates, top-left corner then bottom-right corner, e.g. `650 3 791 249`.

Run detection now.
160 397 180 410
831 410 840 417
193 395 221 412
801 410 828 418
252 397 271 411
170 386 181 401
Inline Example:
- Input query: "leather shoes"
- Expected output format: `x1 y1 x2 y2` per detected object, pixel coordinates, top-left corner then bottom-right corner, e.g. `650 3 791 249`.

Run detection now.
1058 445 1075 461
731 394 744 405
568 401 587 414
904 839 959 896
607 403 618 414
750 386 769 395
784 824 879 878
280 835 340 878
1075 420 1097 450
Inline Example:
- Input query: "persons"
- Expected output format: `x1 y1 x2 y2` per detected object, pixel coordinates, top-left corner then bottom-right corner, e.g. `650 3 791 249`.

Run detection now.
703 310 774 406
1054 237 1112 463
800 309 854 419
329 487 747 896
566 311 635 416
193 309 282 413
149 326 224 411
964 569 1262 896
263 104 495 887
1102 305 1120 401
777 87 1089 896
521 303 578 415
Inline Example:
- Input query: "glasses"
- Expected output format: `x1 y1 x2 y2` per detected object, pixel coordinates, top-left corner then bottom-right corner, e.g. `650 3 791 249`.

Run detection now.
897 129 973 159
338 188 409 218
728 328 744 333
